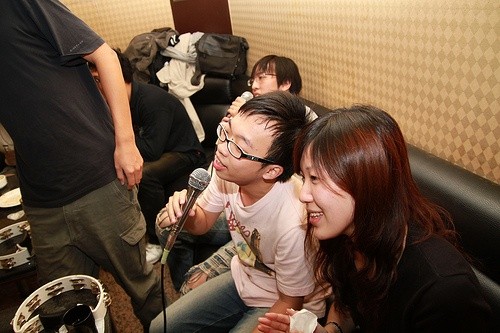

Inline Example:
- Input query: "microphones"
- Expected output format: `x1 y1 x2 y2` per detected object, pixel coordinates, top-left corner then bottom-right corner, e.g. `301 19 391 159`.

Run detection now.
160 167 211 265
226 91 254 119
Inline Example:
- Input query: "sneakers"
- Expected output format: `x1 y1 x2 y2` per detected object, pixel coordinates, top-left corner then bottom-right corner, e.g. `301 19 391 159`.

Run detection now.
145 243 162 264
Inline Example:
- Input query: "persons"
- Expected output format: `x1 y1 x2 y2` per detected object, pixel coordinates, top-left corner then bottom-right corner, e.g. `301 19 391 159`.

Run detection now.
85 47 207 264
258 104 496 333
155 55 320 300
0 0 172 333
149 92 315 332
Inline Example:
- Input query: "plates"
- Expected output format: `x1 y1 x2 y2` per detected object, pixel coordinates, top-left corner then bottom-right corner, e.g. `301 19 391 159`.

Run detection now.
0 187 22 208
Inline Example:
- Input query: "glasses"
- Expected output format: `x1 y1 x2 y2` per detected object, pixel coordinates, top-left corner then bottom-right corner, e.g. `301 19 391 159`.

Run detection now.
216 121 278 165
245 72 275 86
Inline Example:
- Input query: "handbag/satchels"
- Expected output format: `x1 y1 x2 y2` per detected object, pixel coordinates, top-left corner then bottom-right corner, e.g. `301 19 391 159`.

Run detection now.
194 31 247 76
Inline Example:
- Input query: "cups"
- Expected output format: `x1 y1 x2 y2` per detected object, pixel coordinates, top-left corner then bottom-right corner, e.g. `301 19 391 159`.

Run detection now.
62 303 98 333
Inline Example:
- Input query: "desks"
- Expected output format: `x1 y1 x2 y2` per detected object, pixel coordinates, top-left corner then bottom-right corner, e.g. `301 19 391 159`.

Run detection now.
0 165 118 333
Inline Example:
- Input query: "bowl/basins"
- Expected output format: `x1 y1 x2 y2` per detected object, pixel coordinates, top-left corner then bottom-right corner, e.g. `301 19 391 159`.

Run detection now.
0 175 8 189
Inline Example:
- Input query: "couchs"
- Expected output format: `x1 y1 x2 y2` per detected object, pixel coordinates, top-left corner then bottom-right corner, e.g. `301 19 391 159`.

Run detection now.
189 78 500 333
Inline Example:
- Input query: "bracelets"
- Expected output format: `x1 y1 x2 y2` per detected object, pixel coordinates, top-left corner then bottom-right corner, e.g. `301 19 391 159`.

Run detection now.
326 322 340 330
